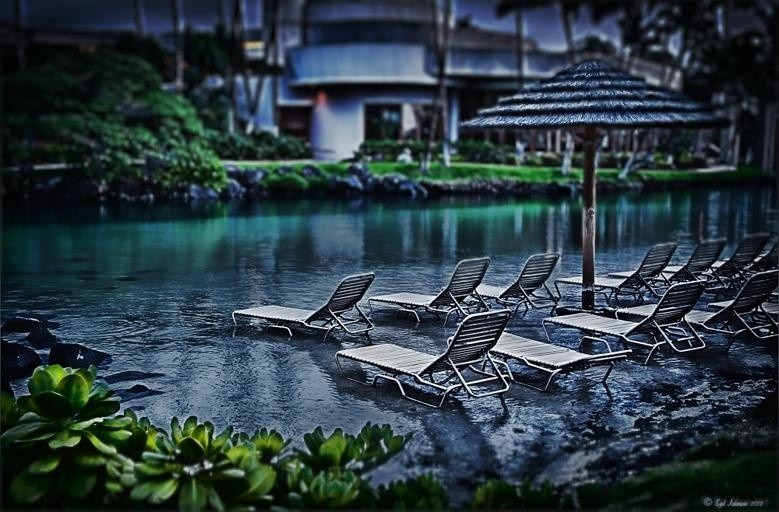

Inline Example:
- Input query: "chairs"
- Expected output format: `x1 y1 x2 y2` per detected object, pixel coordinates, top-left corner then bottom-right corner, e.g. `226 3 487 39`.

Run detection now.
232 232 779 408
